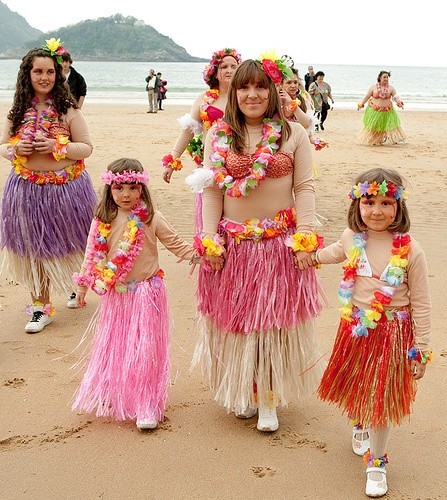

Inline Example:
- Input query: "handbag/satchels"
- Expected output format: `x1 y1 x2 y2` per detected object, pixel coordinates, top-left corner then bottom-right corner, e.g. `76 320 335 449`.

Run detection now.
148 76 156 89
322 101 330 110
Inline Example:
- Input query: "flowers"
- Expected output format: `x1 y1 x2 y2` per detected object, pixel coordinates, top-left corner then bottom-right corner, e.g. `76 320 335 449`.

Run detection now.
256 49 294 84
351 418 369 429
103 170 148 186
370 99 393 111
25 302 56 318
12 159 85 186
253 389 283 411
218 207 297 244
342 309 409 322
203 48 242 84
113 269 164 293
362 449 389 467
349 182 411 202
43 37 68 65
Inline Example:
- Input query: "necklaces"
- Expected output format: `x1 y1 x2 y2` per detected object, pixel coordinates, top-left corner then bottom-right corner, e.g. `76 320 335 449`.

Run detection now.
201 89 220 131
89 201 144 296
208 114 282 197
20 96 56 142
338 231 411 338
377 83 389 99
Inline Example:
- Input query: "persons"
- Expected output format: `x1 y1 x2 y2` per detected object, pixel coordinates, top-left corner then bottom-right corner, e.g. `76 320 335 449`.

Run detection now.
144 68 171 113
304 65 334 131
75 158 224 432
200 59 317 432
355 71 407 147
294 168 431 498
0 46 98 333
163 47 330 184
53 52 89 110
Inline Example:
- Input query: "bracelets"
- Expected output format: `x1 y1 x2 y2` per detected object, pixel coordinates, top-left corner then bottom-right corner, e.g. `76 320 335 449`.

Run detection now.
52 134 71 162
397 101 404 108
194 233 226 255
357 103 364 108
286 98 301 112
407 346 435 365
162 154 183 171
291 231 325 253
7 135 28 165
71 271 85 285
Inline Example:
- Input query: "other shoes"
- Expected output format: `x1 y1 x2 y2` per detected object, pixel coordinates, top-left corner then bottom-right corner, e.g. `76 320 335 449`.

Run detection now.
151 112 157 113
366 467 387 497
315 125 319 131
158 109 163 110
352 425 371 454
147 111 152 113
136 408 157 428
320 124 324 130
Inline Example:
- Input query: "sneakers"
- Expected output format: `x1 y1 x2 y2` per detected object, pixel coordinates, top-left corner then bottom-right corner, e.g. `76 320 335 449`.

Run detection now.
257 399 279 431
67 293 82 307
25 310 52 332
235 398 257 418
